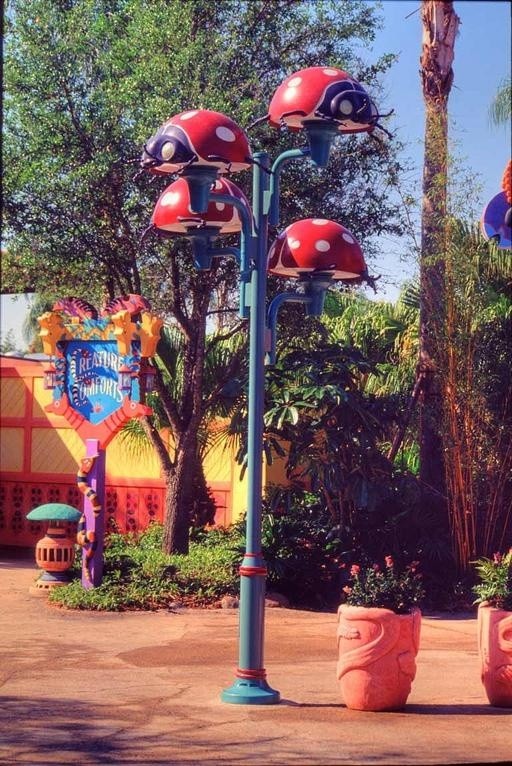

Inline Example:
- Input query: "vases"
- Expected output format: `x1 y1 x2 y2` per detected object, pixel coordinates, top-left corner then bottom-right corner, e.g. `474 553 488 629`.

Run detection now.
477 604 512 709
336 602 422 712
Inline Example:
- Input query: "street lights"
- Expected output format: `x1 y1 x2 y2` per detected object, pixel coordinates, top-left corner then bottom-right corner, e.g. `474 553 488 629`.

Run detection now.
119 65 395 706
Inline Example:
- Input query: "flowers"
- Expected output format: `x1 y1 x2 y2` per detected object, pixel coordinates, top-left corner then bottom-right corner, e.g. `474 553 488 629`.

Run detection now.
469 549 512 612
338 556 428 614
